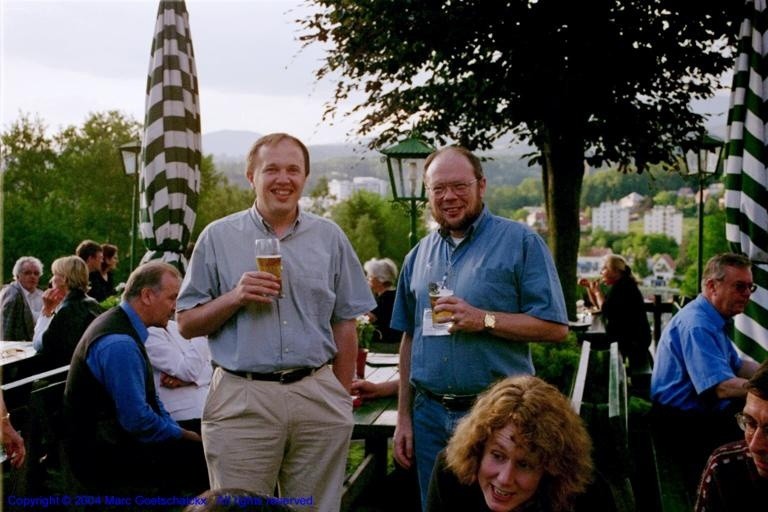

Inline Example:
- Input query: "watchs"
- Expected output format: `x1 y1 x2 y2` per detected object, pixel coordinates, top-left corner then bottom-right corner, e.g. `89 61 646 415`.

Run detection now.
482 309 496 333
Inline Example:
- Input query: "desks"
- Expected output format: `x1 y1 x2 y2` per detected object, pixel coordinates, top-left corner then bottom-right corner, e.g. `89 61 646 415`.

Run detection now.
340 352 401 505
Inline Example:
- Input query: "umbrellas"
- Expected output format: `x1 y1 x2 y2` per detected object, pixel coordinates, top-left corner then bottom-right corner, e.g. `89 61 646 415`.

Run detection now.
723 0 768 371
138 0 201 278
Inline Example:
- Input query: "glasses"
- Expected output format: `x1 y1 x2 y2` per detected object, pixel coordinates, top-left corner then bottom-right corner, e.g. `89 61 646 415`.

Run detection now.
718 281 760 295
735 413 768 440
428 179 477 197
18 270 42 278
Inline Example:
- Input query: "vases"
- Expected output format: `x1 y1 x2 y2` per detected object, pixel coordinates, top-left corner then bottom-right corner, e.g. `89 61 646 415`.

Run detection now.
355 347 368 380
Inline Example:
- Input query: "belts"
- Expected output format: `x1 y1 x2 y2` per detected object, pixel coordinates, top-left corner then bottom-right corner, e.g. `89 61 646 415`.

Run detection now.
210 360 331 384
412 384 481 411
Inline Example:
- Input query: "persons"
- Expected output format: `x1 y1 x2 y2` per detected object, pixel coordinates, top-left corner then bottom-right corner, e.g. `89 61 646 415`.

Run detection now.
389 146 570 512
174 132 377 512
576 255 651 364
694 359 768 511
139 299 214 443
650 252 761 476
71 241 123 302
350 379 400 401
0 253 45 341
424 374 615 511
57 260 210 491
655 276 667 286
1 391 27 469
27 255 106 369
362 256 405 345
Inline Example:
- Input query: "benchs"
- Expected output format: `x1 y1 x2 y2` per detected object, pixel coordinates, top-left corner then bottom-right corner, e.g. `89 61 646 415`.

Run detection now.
565 338 592 421
608 340 696 511
0 363 70 451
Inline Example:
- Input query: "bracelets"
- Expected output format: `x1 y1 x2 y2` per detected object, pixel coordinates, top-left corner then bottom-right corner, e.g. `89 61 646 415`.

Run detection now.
594 290 601 296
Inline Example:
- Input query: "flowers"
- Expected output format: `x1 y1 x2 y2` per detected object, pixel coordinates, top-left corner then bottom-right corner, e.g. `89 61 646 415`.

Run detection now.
354 314 376 351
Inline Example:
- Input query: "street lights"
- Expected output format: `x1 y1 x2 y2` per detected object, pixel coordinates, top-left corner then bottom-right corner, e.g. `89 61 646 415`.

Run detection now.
378 120 441 255
678 124 726 298
115 134 143 276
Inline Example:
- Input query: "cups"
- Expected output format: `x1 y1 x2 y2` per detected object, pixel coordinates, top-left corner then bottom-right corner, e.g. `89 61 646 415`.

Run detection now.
425 259 458 331
254 237 285 300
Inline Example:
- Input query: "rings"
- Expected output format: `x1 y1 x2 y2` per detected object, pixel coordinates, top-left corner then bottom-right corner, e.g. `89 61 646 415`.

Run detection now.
451 314 455 322
